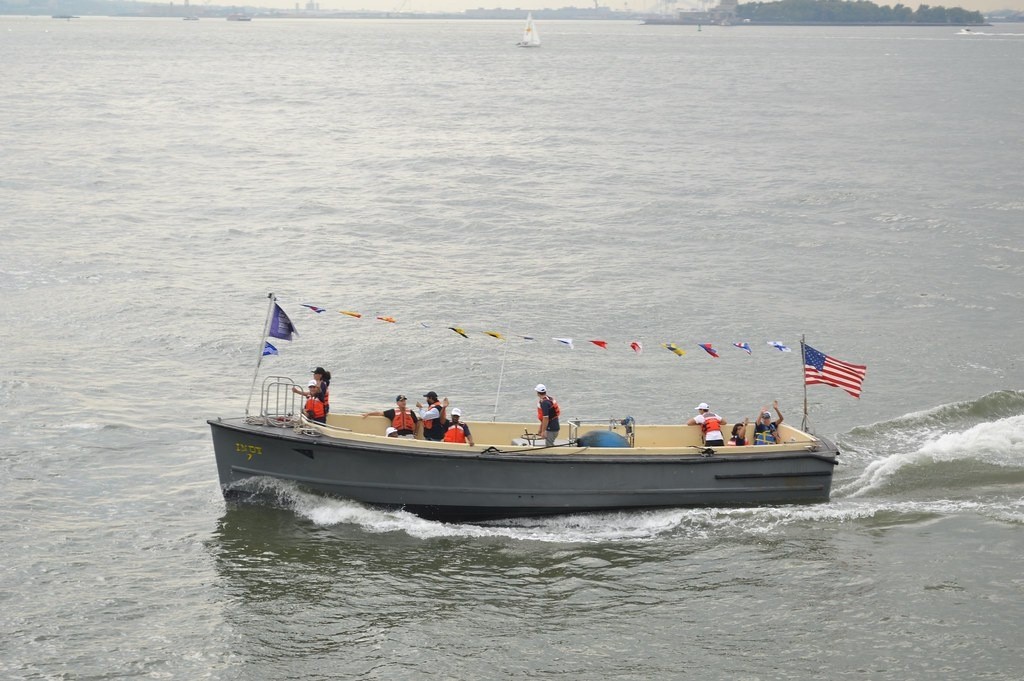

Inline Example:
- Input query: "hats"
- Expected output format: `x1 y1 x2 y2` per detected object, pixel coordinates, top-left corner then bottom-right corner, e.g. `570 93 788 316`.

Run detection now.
695 403 709 409
311 368 325 374
534 384 546 393
386 426 397 438
762 411 771 418
308 380 316 387
396 395 407 401
451 408 461 416
423 392 438 398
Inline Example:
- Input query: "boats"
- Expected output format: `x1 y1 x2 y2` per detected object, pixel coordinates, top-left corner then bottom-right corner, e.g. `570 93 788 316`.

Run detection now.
52 14 73 18
184 16 199 20
205 375 842 525
226 13 252 21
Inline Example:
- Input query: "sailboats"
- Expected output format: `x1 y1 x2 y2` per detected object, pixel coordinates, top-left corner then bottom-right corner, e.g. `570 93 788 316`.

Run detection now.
518 11 541 47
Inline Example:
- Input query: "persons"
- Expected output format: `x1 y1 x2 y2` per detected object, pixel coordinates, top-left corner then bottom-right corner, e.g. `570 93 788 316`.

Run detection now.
361 395 418 438
303 379 326 423
416 391 443 442
730 418 749 446
440 398 474 447
687 403 727 446
386 427 398 438
754 401 783 445
534 384 560 446
292 367 331 414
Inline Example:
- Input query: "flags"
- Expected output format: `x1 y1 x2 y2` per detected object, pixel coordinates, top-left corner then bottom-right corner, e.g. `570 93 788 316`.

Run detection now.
302 304 642 354
734 343 751 355
699 344 719 357
269 303 299 342
801 343 866 400
663 343 684 357
767 341 791 353
